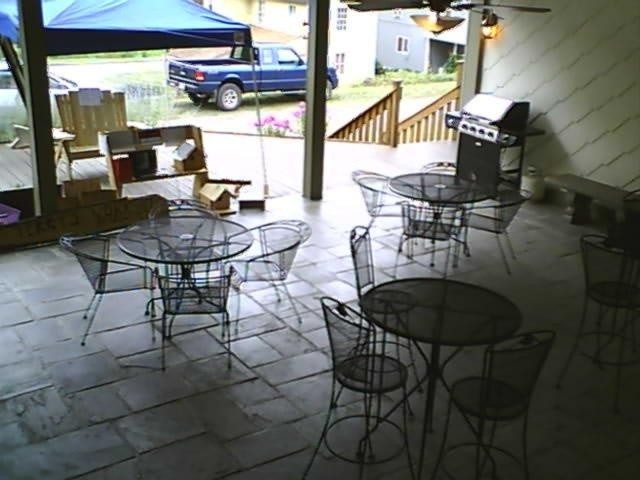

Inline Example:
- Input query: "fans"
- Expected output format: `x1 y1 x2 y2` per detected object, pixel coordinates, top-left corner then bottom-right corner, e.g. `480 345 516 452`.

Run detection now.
341 0 551 36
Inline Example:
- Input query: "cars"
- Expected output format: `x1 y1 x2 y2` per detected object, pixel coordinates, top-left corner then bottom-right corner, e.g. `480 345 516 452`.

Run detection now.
0 58 81 145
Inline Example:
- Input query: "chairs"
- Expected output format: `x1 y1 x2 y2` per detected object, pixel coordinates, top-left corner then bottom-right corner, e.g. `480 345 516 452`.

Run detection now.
353 160 535 276
556 189 640 415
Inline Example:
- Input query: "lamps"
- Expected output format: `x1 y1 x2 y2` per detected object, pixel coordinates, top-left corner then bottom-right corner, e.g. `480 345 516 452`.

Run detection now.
410 12 464 34
480 10 501 37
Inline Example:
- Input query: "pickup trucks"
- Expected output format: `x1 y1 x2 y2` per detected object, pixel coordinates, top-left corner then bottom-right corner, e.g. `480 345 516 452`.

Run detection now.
167 41 338 111
